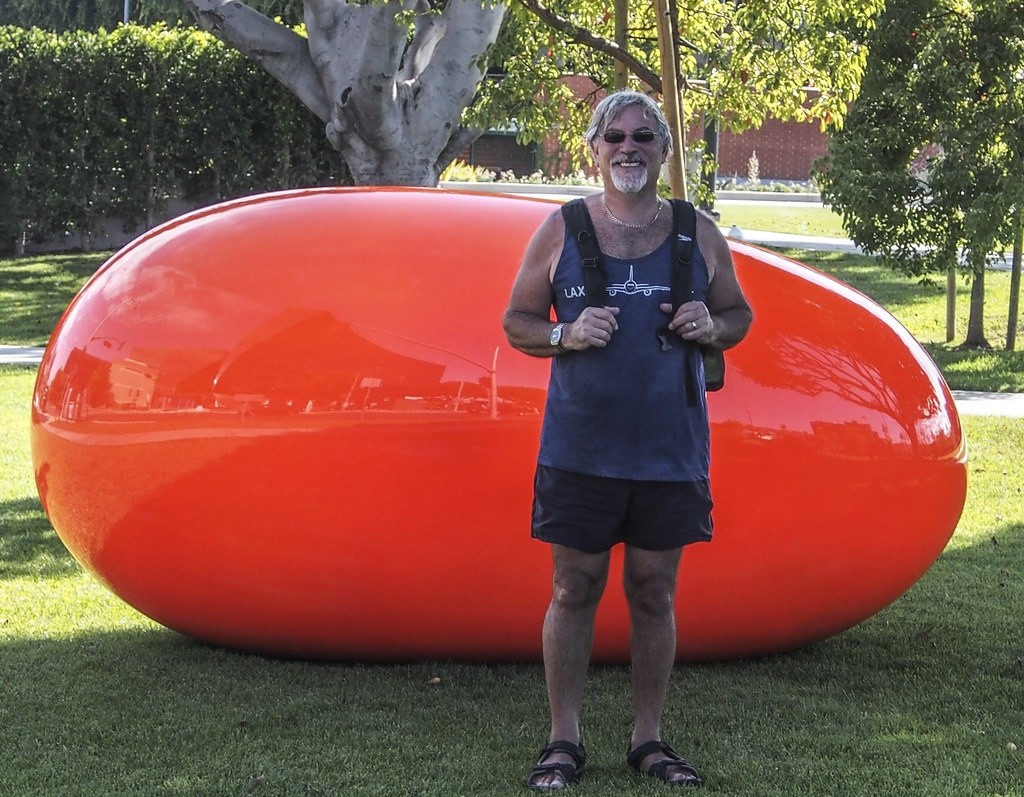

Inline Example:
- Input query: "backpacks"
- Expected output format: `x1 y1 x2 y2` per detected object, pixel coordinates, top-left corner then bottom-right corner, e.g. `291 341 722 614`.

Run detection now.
561 198 725 392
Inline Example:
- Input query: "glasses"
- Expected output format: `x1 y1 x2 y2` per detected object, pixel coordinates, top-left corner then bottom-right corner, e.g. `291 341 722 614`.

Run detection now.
594 126 662 143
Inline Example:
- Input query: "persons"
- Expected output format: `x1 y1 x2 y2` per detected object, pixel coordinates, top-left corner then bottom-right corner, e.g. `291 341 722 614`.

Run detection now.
502 89 754 790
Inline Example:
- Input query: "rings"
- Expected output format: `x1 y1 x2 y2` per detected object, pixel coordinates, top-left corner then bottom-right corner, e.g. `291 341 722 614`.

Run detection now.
691 321 697 330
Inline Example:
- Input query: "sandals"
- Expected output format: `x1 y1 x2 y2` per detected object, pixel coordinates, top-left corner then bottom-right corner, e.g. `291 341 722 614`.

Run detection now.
626 737 704 788
527 741 589 792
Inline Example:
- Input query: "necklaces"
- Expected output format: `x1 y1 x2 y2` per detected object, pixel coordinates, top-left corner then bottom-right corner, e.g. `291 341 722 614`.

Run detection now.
600 194 663 230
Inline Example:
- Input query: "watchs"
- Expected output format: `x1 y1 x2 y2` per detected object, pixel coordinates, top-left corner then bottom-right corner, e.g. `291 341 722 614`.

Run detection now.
549 322 572 353
